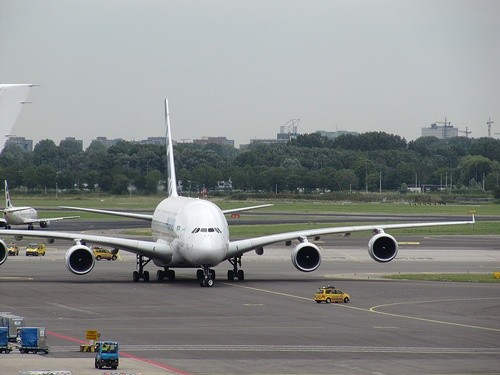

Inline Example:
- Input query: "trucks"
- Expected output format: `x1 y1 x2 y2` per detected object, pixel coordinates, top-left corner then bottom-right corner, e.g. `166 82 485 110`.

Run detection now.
94 340 119 369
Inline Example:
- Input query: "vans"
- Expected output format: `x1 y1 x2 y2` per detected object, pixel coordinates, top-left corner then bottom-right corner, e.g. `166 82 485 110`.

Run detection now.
26 241 46 256
92 246 116 261
6 242 18 256
1 178 80 231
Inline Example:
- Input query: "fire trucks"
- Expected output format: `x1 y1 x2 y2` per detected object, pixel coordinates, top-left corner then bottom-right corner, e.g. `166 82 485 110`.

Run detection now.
314 285 351 304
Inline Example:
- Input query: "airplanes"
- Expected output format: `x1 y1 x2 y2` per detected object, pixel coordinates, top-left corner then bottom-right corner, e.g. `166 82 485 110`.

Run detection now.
0 100 479 286
